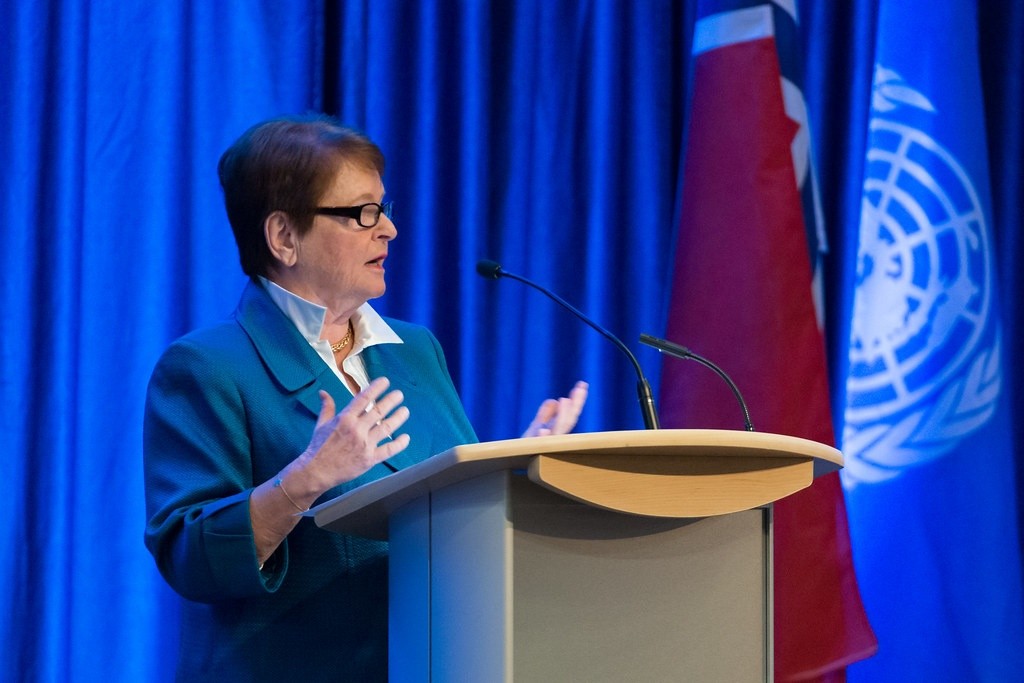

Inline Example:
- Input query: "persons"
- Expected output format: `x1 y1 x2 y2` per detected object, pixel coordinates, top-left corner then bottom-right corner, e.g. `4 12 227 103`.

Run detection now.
145 110 590 683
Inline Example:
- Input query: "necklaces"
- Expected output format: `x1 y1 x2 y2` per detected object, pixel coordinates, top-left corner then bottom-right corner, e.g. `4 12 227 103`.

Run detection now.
330 325 351 352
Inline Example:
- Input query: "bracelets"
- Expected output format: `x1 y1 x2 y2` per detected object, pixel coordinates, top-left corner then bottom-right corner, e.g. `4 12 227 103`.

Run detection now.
274 471 310 512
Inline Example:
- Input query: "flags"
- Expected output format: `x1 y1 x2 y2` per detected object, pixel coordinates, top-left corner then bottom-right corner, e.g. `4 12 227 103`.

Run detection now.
664 0 1023 683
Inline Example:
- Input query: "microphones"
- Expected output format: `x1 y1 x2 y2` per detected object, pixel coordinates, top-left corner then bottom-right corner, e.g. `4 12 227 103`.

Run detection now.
639 333 754 432
477 259 660 430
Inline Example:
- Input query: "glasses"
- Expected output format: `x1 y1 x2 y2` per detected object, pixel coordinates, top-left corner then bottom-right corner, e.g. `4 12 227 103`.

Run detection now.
309 202 394 228
